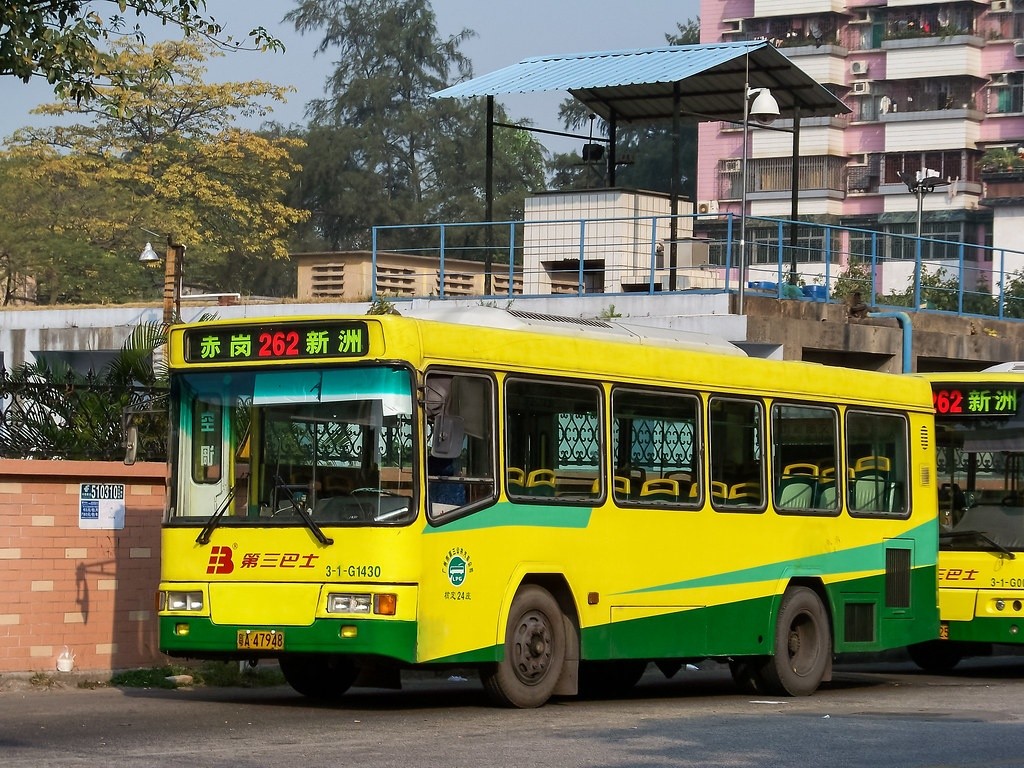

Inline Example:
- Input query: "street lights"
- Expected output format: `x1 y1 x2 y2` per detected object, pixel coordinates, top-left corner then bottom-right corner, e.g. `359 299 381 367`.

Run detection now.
138 231 184 325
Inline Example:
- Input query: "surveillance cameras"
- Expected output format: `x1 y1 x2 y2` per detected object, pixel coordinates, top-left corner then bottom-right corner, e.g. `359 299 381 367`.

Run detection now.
749 89 780 125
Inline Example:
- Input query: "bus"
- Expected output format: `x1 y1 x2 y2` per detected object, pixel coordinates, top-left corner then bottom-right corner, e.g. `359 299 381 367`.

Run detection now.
117 303 941 710
899 360 1024 674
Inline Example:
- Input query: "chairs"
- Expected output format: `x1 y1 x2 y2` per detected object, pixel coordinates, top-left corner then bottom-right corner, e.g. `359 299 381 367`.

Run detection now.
285 454 906 513
938 483 1024 528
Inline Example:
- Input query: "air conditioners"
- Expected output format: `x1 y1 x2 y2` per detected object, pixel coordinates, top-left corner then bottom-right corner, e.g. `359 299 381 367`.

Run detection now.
697 200 719 219
850 60 868 74
991 1 1008 11
989 73 1009 85
852 82 871 94
1013 43 1024 58
726 161 741 172
